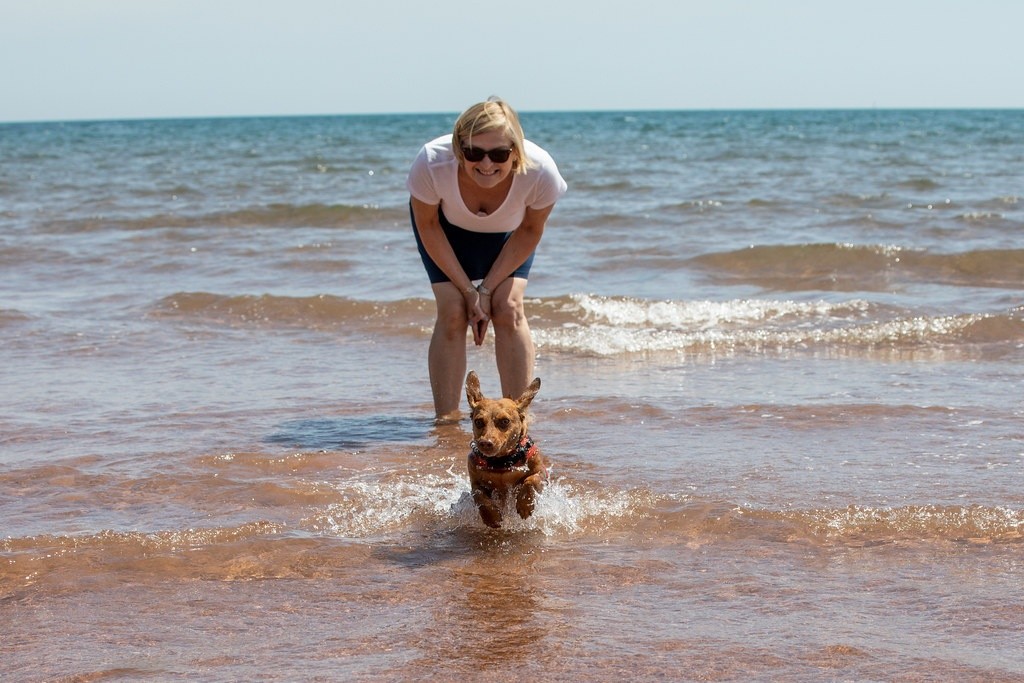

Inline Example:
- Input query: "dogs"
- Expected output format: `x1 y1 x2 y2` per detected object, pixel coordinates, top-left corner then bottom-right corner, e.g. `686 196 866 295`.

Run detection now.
465 371 549 529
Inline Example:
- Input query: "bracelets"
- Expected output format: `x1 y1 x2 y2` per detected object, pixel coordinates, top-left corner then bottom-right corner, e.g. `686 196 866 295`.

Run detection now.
461 287 477 294
477 285 492 295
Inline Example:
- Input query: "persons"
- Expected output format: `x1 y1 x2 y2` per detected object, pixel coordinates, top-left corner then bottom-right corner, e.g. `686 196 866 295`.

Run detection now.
406 96 567 420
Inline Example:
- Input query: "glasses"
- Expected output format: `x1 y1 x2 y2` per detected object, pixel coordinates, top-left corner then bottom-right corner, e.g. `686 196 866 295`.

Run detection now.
462 143 514 163
